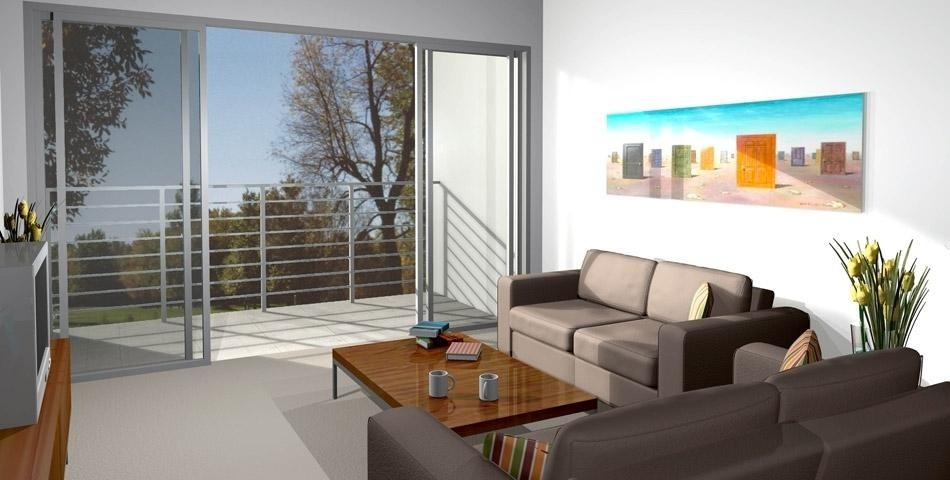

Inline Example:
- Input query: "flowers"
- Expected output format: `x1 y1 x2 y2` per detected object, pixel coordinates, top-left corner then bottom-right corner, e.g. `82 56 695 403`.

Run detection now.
827 232 932 353
0 193 57 243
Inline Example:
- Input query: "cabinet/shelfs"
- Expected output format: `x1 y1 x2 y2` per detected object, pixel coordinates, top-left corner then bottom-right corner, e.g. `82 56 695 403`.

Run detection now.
1 336 75 480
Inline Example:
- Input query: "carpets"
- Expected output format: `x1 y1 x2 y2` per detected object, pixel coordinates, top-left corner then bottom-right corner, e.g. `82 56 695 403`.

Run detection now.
274 392 391 479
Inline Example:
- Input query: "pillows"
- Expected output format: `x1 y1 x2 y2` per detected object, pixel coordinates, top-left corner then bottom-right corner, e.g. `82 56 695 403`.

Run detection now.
779 327 822 372
481 429 550 480
687 278 715 323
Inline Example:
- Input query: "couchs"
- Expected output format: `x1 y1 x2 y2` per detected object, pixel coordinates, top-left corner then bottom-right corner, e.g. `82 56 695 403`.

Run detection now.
365 348 950 480
496 246 812 406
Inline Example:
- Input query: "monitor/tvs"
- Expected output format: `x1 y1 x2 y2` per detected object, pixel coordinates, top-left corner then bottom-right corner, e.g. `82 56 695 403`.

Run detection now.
0 240 52 430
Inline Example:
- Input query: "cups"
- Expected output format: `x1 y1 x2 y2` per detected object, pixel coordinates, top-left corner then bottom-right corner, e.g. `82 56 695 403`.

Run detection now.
477 372 499 402
430 369 456 400
